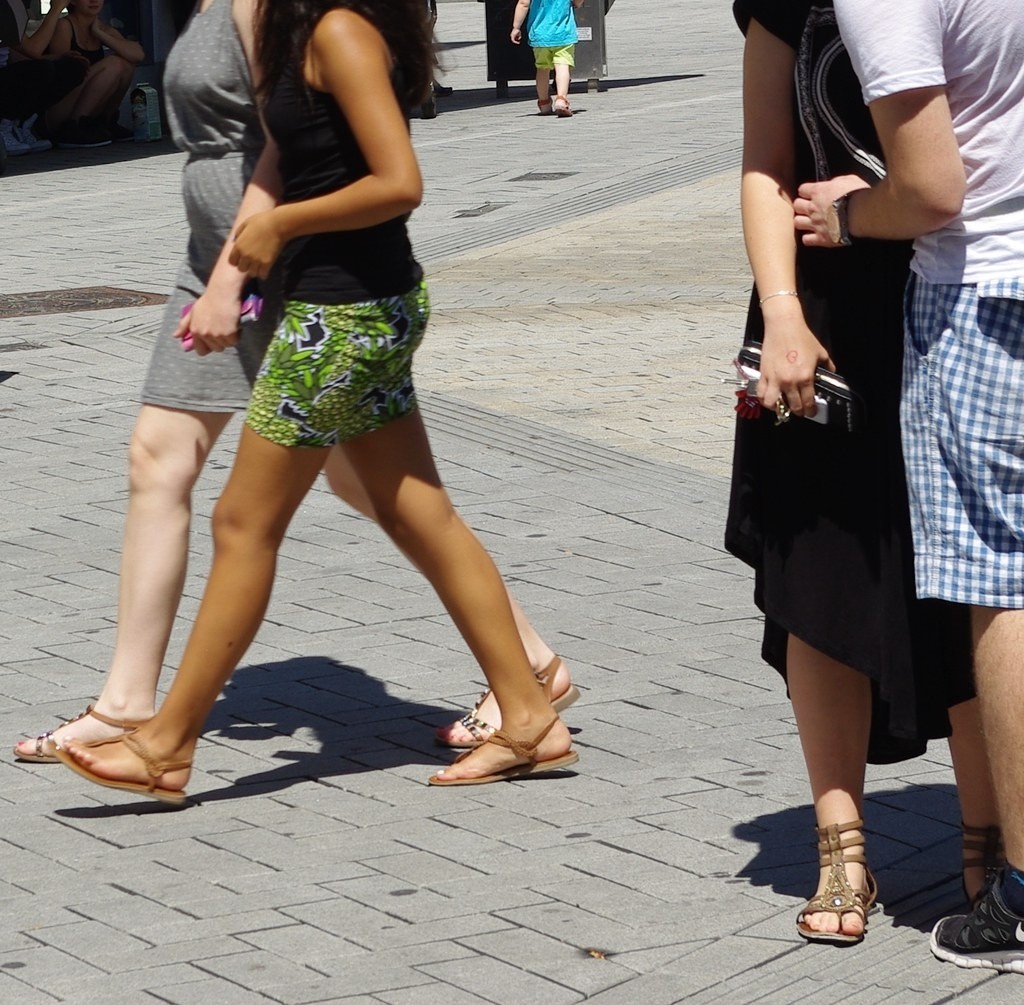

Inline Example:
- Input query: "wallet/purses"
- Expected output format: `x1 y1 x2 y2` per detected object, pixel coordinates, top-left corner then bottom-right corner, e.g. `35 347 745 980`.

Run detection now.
723 340 853 435
180 292 264 351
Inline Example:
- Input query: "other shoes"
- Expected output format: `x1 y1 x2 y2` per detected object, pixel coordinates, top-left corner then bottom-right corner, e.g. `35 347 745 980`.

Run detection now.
552 95 572 117
537 98 553 115
97 116 136 141
433 85 454 96
47 116 112 148
420 102 437 120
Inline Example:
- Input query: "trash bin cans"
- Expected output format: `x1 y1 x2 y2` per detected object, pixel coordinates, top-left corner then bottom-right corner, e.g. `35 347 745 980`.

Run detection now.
479 0 609 99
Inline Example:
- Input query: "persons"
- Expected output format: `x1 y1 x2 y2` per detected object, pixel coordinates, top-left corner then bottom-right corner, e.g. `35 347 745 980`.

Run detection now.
725 0 1024 970
0 0 581 803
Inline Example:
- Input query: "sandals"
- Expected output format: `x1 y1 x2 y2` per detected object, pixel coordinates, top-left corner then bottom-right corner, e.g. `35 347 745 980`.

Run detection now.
428 715 579 786
796 818 877 940
12 704 157 763
435 656 580 746
48 729 193 806
960 821 1009 913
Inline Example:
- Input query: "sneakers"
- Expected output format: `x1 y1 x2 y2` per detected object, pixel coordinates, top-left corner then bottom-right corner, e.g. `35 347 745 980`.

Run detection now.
0 118 32 156
929 868 1024 975
14 113 52 152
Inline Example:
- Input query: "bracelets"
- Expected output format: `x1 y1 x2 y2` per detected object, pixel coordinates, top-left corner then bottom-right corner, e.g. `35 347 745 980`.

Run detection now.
758 290 798 305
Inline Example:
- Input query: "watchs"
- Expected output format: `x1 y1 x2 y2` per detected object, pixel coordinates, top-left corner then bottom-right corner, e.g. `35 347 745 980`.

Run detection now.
823 188 859 247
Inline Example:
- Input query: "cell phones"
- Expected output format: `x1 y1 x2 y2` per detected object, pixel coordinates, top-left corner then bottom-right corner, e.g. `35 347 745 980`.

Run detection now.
739 366 829 425
